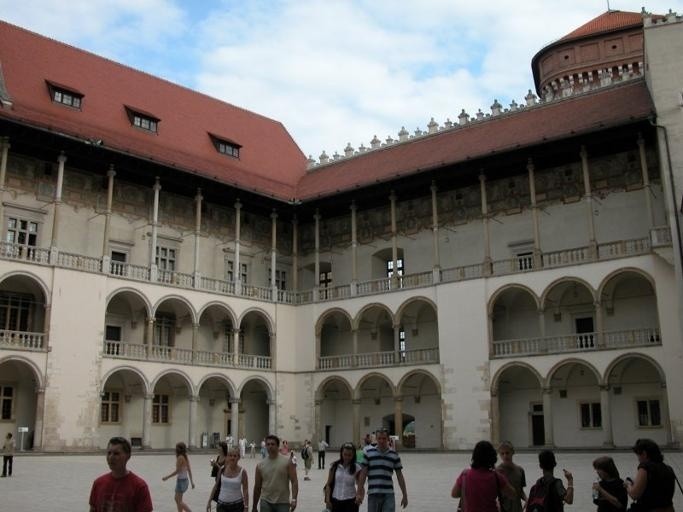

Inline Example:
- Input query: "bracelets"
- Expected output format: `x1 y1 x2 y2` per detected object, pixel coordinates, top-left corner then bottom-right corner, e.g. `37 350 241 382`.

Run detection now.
290 498 297 502
603 492 609 498
243 505 248 508
566 484 574 490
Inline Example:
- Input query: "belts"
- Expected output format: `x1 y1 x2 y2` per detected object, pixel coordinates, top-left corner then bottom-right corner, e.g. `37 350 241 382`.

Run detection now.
176 478 188 482
216 499 242 505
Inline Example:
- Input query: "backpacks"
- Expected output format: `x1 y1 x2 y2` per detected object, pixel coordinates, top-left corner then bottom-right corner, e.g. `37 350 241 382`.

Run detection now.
301 448 308 460
526 487 557 512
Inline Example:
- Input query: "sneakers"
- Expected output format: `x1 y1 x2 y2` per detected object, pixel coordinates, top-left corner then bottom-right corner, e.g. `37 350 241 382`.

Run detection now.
304 477 311 481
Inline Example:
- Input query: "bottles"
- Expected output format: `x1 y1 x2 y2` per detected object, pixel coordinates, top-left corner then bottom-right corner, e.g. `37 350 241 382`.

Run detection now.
592 477 600 499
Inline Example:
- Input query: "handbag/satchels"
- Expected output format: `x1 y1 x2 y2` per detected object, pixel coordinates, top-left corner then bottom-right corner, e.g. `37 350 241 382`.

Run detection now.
210 464 216 478
212 482 221 501
324 480 334 502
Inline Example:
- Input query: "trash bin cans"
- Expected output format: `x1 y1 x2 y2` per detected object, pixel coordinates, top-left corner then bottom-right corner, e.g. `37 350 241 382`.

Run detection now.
202 432 208 448
213 432 220 449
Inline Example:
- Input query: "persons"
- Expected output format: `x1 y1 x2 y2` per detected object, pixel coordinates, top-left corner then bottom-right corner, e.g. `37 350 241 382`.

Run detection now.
590 455 628 511
354 427 407 511
325 441 365 512
494 439 529 511
362 429 396 452
198 431 333 483
622 437 675 511
87 435 153 511
352 441 366 486
451 439 517 512
524 449 574 512
250 434 298 512
205 445 248 512
161 441 194 512
0 432 15 477
